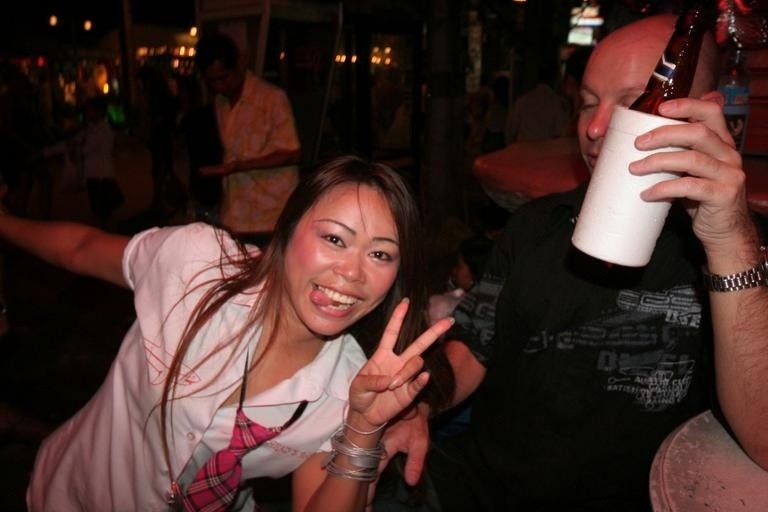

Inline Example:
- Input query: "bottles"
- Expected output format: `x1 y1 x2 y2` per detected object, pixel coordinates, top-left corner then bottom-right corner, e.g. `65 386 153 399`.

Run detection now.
714 39 752 155
570 0 714 270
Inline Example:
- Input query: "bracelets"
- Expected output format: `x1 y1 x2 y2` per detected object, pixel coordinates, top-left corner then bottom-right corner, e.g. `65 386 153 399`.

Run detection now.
701 247 768 293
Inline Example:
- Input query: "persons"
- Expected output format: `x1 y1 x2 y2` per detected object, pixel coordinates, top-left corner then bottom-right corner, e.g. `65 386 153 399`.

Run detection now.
367 14 768 512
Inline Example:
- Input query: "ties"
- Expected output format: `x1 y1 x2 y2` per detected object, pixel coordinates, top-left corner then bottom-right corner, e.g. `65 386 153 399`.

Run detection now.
180 408 281 512
